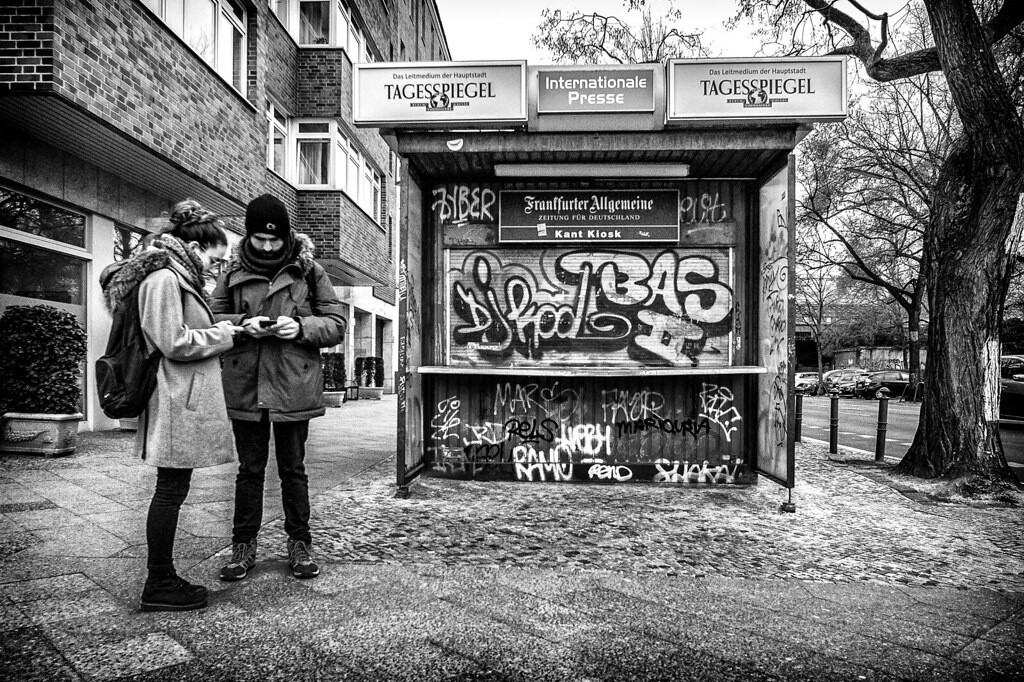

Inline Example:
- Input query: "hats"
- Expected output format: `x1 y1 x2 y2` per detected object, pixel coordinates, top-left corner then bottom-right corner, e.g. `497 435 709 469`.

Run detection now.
245 194 289 242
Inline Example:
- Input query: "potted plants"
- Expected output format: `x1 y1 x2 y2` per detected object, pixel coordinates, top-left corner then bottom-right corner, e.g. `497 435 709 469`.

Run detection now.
354 357 385 400
321 352 346 407
0 304 88 456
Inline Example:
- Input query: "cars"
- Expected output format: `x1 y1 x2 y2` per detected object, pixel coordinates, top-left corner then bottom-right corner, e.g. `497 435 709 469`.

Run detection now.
795 372 826 396
815 368 871 398
1001 356 1024 421
854 371 912 400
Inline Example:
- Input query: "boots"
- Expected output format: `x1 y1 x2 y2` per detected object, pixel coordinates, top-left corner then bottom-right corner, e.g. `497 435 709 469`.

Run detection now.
172 569 208 596
139 568 208 610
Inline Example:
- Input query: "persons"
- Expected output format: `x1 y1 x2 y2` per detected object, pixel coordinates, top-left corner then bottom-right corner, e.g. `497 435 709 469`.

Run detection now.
220 195 348 581
99 198 247 610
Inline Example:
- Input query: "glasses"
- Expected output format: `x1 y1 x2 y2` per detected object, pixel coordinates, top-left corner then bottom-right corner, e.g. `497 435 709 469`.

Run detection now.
187 240 220 269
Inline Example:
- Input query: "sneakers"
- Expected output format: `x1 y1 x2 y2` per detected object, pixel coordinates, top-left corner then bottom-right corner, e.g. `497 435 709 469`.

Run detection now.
219 538 258 580
287 537 321 578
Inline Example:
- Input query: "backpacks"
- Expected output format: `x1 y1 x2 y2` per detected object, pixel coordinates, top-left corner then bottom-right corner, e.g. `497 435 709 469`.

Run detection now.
95 267 186 419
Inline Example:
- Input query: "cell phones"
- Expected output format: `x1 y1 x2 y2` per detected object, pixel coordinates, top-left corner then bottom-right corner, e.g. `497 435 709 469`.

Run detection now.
259 319 277 328
235 322 250 333
